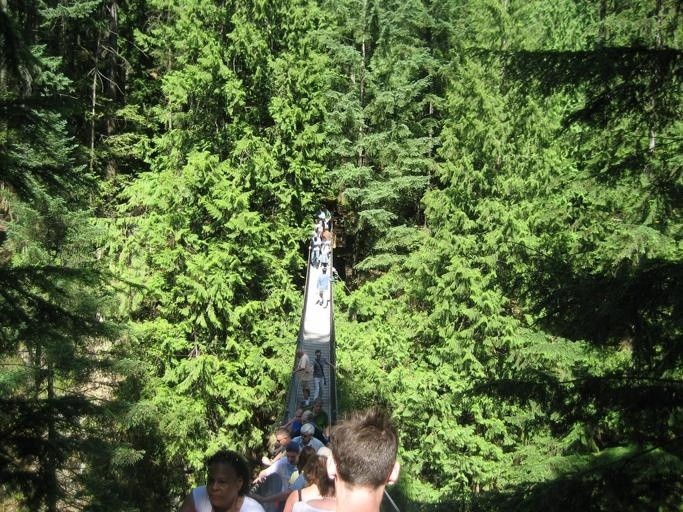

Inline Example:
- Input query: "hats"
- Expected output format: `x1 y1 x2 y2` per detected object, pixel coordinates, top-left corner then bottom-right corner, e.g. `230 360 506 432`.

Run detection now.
285 441 300 454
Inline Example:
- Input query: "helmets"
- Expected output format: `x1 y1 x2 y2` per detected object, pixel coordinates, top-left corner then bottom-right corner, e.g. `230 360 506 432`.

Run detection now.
300 423 316 435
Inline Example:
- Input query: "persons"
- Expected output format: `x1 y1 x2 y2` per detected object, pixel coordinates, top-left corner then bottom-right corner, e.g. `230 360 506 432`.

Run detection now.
308 202 334 309
179 345 403 512
